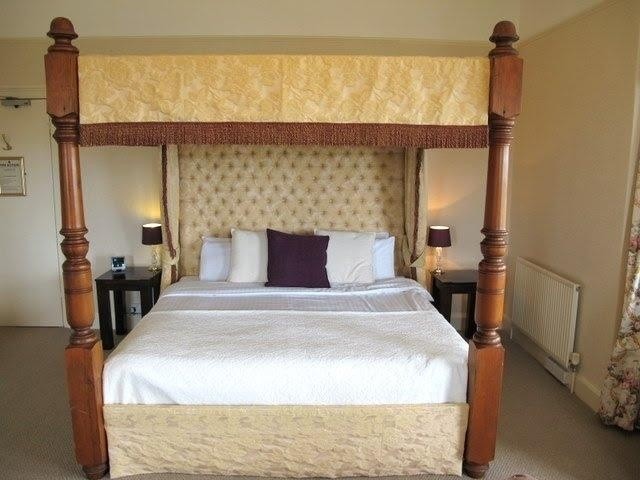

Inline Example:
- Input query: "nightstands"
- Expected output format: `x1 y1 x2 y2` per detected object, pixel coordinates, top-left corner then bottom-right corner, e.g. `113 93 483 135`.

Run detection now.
428 265 482 339
92 263 162 350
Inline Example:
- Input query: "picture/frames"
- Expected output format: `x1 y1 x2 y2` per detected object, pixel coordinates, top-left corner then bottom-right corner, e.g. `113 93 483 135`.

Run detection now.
0 156 27 198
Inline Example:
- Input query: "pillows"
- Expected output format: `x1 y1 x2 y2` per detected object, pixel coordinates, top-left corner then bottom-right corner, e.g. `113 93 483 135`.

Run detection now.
369 236 398 281
224 226 264 284
264 226 328 291
310 227 377 288
196 233 232 283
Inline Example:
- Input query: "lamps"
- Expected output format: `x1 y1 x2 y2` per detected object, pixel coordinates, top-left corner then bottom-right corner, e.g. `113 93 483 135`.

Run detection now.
424 221 451 276
138 220 163 271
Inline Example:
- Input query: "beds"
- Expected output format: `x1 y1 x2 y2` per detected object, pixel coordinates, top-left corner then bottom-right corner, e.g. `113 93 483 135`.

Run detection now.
40 8 525 476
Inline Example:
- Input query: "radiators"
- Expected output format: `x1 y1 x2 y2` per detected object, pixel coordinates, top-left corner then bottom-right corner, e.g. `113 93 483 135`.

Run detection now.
508 253 584 395
